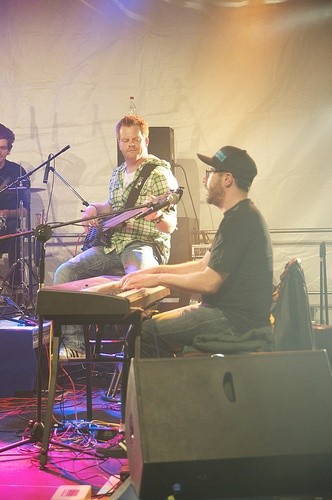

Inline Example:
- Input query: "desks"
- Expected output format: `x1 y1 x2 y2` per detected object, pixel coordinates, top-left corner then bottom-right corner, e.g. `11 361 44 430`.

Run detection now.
38 298 164 470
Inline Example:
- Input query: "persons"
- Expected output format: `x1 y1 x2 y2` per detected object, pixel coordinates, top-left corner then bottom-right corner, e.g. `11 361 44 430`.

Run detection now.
0 123 30 287
53 114 178 359
121 144 273 360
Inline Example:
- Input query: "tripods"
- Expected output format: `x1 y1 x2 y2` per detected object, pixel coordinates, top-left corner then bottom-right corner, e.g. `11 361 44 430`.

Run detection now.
0 190 156 460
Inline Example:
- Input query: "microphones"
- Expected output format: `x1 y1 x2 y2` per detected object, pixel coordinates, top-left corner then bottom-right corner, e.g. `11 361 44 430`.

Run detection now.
136 193 179 220
43 154 51 183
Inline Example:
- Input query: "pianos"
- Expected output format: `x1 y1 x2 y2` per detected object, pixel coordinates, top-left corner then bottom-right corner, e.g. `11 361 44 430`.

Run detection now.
36 274 171 471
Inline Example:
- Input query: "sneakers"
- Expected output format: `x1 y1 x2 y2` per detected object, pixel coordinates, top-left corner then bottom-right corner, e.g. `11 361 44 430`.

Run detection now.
52 347 87 359
97 435 127 456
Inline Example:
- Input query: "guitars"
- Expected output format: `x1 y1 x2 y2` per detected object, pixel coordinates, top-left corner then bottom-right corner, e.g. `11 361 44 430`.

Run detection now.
81 186 185 252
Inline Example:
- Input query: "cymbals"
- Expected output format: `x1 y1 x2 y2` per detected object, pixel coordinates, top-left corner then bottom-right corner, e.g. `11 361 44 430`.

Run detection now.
3 186 46 192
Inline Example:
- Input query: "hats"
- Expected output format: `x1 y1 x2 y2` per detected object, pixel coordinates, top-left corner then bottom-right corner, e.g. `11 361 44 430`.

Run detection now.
198 146 258 185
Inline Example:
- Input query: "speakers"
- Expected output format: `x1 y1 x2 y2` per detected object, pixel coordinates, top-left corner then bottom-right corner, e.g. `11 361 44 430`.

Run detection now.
116 127 199 314
125 350 332 500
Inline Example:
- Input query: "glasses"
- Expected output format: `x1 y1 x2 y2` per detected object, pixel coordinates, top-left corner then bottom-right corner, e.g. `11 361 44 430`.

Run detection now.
206 169 226 178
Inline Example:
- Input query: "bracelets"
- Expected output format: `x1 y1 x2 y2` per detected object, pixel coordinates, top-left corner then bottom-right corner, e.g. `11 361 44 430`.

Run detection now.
90 204 96 208
153 214 164 223
